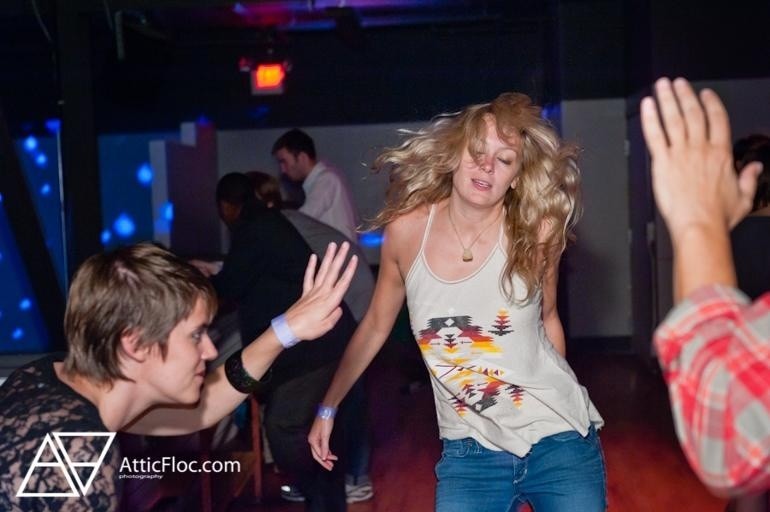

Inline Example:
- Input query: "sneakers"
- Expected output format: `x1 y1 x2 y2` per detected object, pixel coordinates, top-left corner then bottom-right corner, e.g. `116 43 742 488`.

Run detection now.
280 480 306 502
341 479 375 504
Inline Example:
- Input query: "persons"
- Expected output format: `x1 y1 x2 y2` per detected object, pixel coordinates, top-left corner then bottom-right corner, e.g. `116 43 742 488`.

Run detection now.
307 89 620 512
1 235 364 512
718 130 770 300
638 73 770 512
202 124 379 512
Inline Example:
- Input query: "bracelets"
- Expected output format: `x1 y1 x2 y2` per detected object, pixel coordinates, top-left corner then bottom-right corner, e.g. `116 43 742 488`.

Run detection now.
224 350 274 394
318 401 338 421
270 313 299 348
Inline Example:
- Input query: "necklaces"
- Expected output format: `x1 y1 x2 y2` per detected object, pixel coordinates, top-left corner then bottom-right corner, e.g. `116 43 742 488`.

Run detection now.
447 189 504 266
